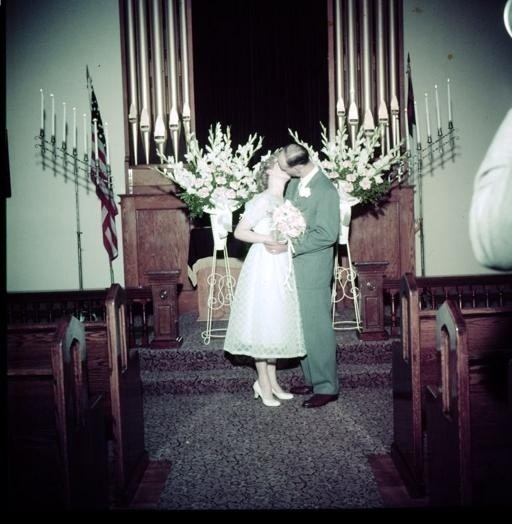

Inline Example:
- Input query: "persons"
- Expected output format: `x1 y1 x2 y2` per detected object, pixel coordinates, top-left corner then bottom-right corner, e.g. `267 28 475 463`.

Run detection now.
468 105 512 272
223 151 309 406
262 142 342 408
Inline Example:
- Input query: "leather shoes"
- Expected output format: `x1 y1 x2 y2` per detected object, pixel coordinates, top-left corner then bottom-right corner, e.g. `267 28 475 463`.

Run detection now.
290 385 313 395
302 394 339 408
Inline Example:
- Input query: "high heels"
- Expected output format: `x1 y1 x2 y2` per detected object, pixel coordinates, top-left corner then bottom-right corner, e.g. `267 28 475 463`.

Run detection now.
252 380 280 406
272 388 293 400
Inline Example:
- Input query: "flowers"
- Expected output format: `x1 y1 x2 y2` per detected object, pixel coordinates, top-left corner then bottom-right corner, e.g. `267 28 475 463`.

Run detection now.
271 199 308 251
153 116 405 221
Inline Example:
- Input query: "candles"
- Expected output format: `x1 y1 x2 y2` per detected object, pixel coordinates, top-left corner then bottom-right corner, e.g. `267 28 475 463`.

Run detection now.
403 76 453 149
38 90 110 165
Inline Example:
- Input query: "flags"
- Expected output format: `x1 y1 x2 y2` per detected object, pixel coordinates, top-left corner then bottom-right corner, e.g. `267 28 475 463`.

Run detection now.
88 85 119 264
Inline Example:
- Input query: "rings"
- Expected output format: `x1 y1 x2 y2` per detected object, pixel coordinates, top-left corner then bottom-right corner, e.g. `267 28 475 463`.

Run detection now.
272 250 275 253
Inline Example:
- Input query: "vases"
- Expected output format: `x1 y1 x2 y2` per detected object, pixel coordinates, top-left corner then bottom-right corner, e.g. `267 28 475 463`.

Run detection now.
210 213 231 251
336 199 354 246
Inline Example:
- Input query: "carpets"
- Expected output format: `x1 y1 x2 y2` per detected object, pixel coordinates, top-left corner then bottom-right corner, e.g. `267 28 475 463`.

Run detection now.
132 311 398 509
